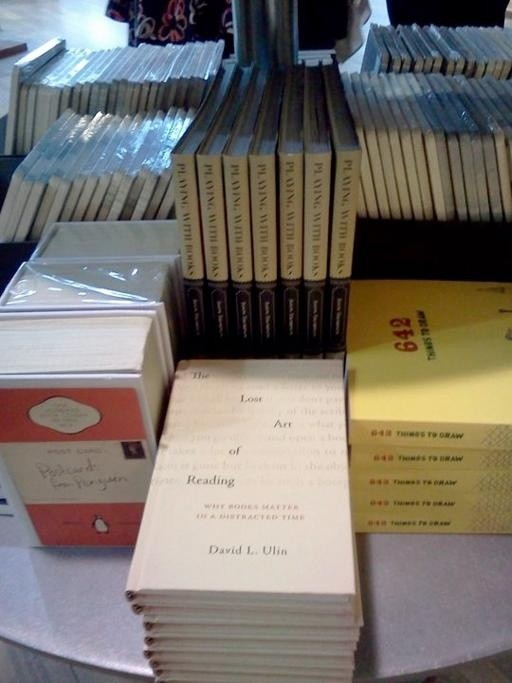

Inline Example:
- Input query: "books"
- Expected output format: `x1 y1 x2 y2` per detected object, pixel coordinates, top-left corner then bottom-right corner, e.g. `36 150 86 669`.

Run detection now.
344 280 511 534
342 22 512 80
6 35 224 158
2 220 182 550
0 109 195 242
169 60 362 358
341 72 512 223
127 358 365 682
